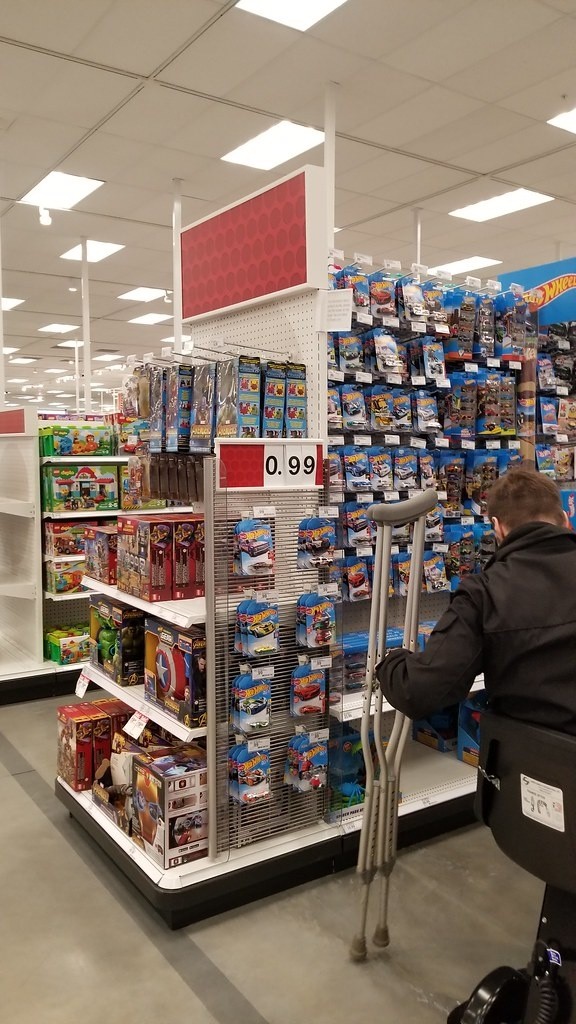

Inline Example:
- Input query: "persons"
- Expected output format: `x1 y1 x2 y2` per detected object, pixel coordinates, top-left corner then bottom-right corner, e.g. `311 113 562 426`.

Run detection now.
377 467 576 738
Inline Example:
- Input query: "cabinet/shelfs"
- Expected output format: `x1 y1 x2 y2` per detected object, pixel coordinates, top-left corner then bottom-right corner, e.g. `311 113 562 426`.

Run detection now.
54 88 576 928
0 405 133 679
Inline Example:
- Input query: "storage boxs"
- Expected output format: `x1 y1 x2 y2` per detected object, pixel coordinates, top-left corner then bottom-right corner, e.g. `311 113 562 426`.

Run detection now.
45 561 52 592
214 512 275 594
410 715 458 753
44 522 52 554
85 526 118 584
74 703 109 788
44 426 111 456
92 699 126 744
149 354 308 448
90 594 145 687
186 512 206 597
47 466 120 512
154 514 196 600
50 561 87 593
57 705 93 791
131 744 210 870
143 618 207 729
109 698 135 724
41 467 50 511
118 516 173 603
50 522 98 556
456 698 483 768
